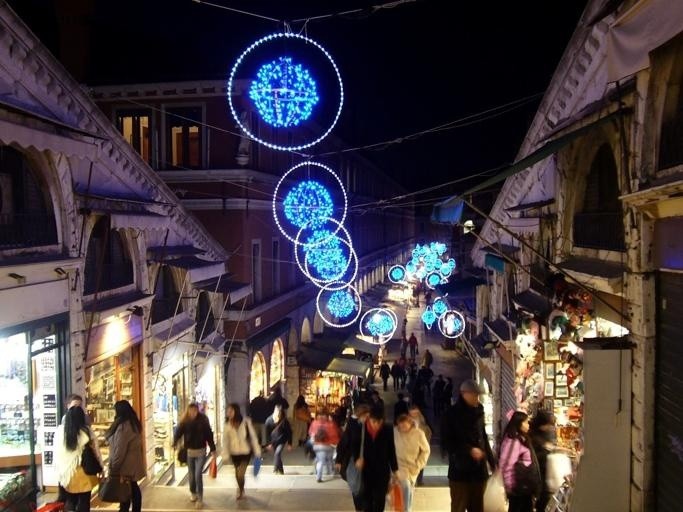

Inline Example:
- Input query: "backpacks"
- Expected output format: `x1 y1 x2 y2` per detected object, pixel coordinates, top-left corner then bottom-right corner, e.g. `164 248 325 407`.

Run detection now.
314 428 328 443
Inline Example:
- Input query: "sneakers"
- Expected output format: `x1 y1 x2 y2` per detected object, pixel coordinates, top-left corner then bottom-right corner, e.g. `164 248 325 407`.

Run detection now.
195 501 202 508
190 495 197 502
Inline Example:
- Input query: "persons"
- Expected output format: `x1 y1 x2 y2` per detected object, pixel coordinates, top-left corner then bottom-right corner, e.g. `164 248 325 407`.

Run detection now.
293 385 433 512
250 391 269 449
268 387 291 419
223 403 262 500
104 400 146 512
59 394 90 507
379 333 453 460
444 380 552 512
265 402 293 475
52 405 104 512
170 403 216 509
413 282 431 308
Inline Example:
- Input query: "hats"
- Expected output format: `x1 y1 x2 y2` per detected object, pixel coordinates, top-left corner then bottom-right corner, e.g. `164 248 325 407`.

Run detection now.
460 380 485 394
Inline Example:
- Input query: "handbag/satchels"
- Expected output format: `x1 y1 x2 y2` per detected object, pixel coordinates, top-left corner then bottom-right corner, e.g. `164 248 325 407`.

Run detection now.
545 454 572 492
346 456 362 494
254 457 262 476
100 478 132 501
177 444 187 463
